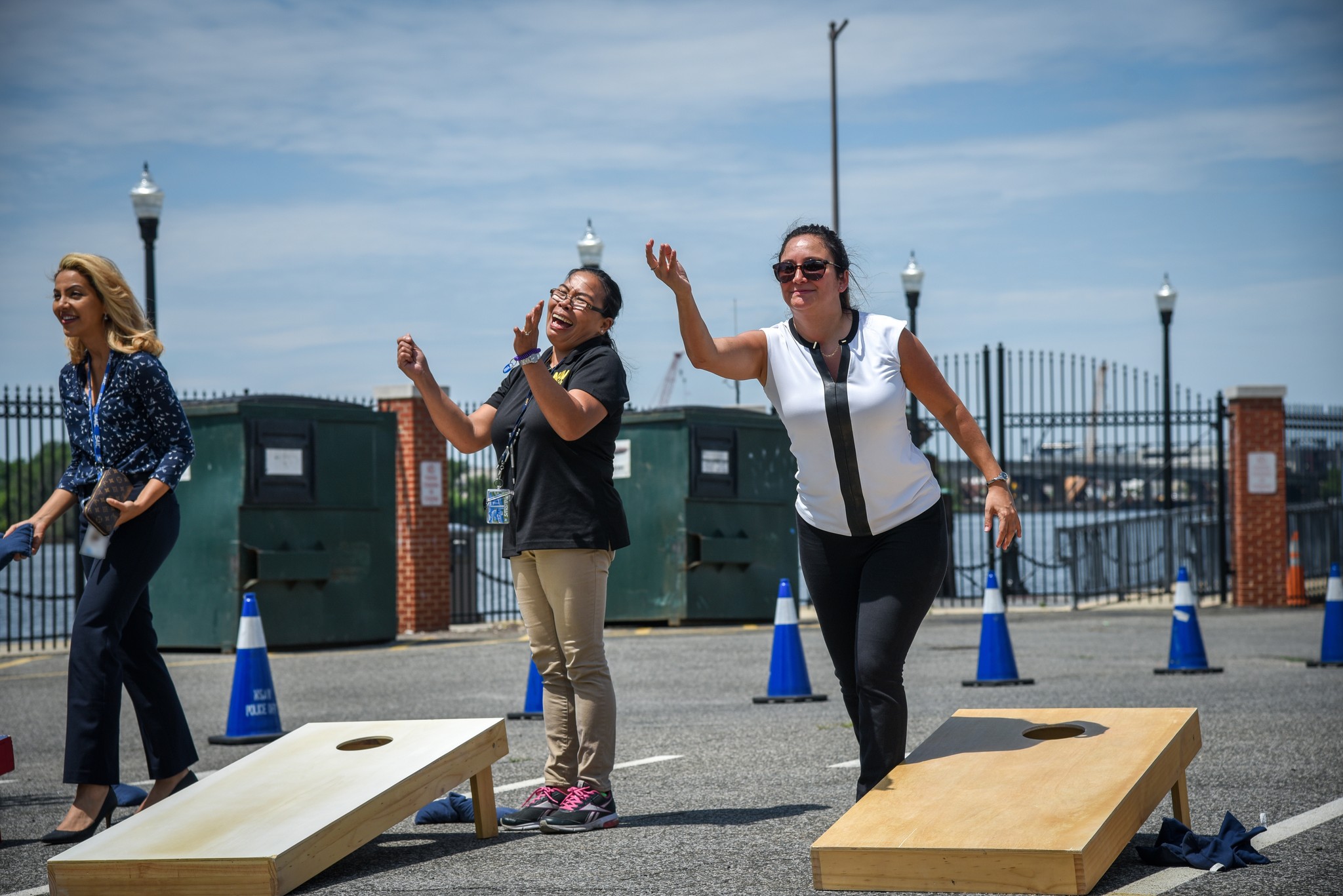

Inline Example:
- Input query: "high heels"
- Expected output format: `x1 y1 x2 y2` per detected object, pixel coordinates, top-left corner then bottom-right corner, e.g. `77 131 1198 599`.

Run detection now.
112 770 199 825
40 783 118 845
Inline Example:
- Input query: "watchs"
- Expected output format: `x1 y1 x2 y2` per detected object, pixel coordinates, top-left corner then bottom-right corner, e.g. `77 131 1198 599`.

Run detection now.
518 351 541 367
984 470 1009 486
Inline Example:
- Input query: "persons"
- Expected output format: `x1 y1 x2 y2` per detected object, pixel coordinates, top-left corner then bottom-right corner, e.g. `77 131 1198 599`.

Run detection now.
646 221 1022 801
4 252 199 843
396 266 630 833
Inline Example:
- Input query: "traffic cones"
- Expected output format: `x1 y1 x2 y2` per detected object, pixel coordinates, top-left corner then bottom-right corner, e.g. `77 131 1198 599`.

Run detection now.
206 591 294 747
1152 564 1223 674
1285 530 1309 608
1302 561 1343 670
960 570 1035 688
507 650 544 720
750 578 828 704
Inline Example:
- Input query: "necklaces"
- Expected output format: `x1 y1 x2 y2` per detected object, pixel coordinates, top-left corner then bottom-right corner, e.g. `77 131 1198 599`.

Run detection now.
545 355 568 370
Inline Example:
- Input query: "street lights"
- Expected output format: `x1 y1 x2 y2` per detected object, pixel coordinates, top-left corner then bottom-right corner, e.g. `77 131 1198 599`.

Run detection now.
1153 272 1179 596
899 248 926 451
129 160 165 340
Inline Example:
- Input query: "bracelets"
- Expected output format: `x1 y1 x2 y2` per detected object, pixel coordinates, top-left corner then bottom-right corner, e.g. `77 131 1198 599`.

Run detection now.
514 348 540 361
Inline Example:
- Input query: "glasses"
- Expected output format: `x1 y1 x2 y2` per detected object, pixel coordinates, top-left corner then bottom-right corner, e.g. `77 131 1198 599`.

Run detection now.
773 259 840 283
549 288 605 315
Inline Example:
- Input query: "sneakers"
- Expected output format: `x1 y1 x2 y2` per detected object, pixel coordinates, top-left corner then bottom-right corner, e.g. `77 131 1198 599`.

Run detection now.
539 780 620 833
499 787 568 830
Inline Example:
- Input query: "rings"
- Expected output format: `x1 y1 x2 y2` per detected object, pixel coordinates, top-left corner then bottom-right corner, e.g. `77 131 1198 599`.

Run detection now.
523 327 533 336
650 264 659 271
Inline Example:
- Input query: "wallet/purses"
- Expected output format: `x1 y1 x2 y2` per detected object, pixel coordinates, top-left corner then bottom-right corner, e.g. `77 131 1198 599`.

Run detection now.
83 467 133 535
